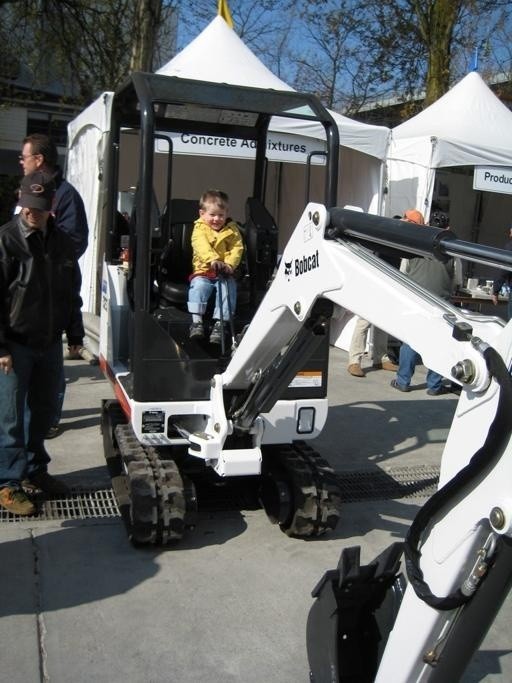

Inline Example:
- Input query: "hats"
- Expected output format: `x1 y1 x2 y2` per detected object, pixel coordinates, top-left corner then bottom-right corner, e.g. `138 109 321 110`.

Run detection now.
402 210 425 225
16 170 56 209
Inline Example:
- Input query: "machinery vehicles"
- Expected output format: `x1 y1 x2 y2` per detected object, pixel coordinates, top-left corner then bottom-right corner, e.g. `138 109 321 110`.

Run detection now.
91 79 507 677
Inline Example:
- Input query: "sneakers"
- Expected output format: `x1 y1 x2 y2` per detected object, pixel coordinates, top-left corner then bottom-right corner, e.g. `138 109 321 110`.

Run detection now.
22 473 70 497
187 322 206 340
0 481 37 516
427 385 445 395
209 321 223 345
390 379 408 392
43 426 61 439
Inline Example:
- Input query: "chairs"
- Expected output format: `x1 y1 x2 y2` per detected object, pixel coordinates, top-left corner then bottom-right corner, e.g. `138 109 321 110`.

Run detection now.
153 199 251 306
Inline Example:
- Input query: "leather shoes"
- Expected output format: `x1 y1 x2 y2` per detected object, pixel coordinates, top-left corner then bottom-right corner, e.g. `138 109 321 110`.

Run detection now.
347 364 364 377
382 362 399 372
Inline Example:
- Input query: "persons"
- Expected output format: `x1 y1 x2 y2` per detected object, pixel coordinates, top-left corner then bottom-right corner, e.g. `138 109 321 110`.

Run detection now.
348 209 512 396
0 173 85 516
11 133 88 259
187 189 246 344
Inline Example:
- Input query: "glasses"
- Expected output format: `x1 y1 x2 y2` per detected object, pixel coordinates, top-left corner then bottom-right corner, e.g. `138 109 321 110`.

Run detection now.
17 153 41 161
